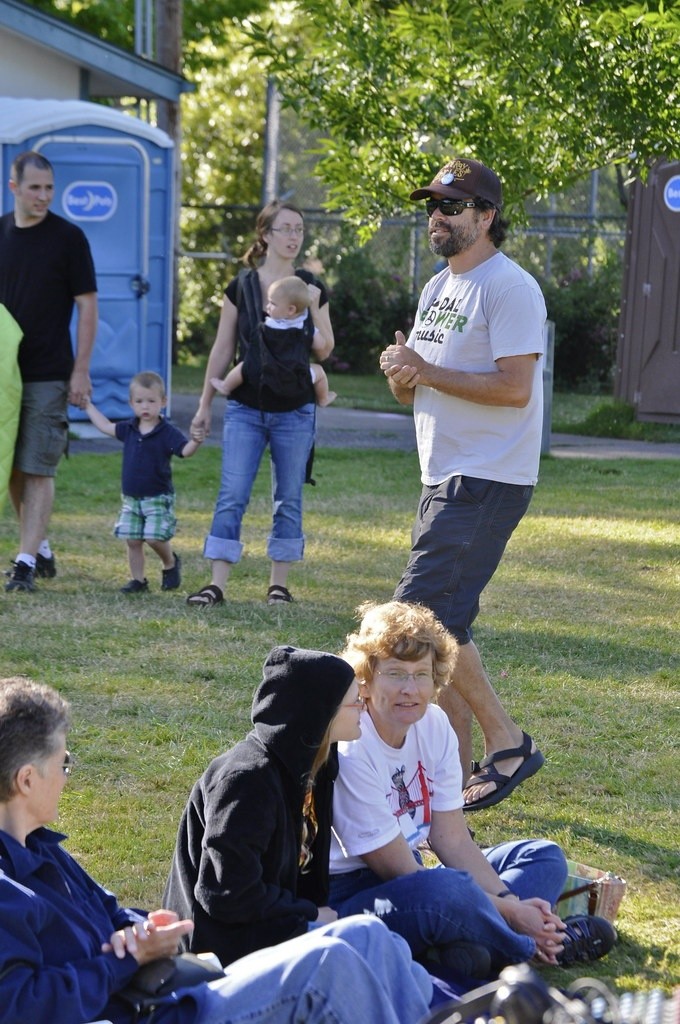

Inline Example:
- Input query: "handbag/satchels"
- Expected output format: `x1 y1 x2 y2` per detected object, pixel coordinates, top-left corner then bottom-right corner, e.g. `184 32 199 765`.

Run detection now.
558 859 626 923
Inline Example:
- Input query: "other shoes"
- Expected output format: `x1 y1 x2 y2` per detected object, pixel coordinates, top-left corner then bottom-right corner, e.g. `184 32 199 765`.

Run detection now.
4 552 56 594
119 577 149 592
160 551 182 590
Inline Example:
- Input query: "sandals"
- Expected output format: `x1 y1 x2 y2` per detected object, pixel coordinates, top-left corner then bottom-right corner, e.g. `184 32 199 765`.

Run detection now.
186 585 223 608
266 585 294 607
462 730 545 811
553 914 619 968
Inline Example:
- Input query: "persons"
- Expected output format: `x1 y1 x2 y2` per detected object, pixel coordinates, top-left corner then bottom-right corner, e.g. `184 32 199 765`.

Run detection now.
162 645 368 970
379 159 547 811
0 677 432 1024
84 372 206 592
0 152 100 592
325 602 618 980
192 198 337 609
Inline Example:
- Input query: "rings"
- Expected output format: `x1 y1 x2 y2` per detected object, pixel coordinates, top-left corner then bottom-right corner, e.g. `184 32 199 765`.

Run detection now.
534 949 544 956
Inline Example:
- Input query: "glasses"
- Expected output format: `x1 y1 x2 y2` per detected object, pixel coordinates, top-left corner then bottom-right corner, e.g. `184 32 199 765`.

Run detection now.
340 696 366 711
272 225 304 234
374 667 438 683
42 750 70 775
424 199 477 217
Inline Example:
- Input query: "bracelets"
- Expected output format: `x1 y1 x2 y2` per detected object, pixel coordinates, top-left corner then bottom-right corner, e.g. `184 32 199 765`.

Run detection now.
498 889 515 897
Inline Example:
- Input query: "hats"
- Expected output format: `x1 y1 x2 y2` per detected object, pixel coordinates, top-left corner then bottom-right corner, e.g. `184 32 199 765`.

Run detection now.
409 158 503 211
